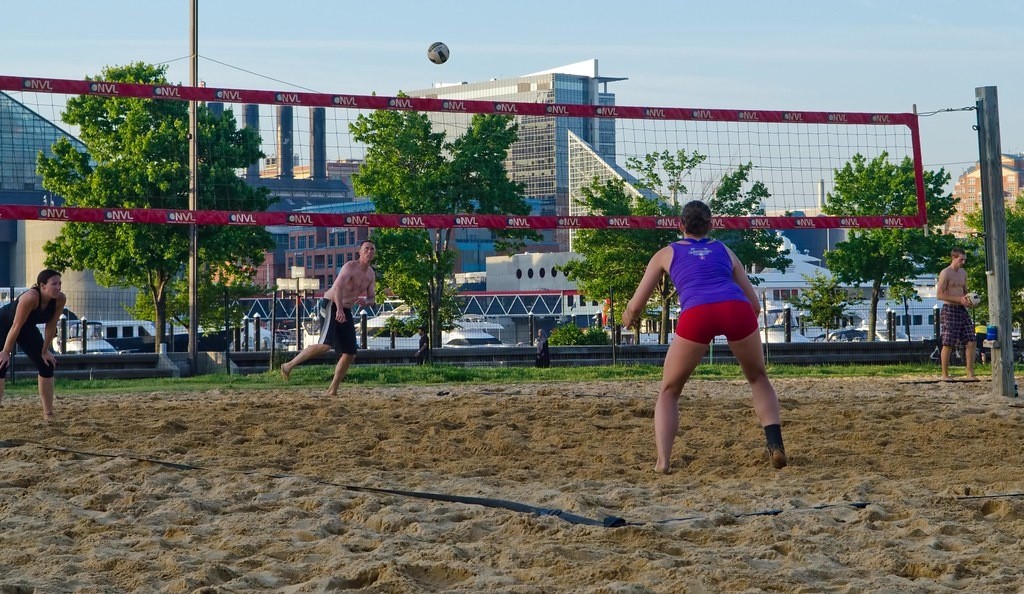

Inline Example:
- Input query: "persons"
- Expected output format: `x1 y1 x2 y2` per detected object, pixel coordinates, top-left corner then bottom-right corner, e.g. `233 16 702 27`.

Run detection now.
0 269 67 422
537 330 549 368
933 248 992 379
413 327 430 365
281 240 376 398
624 201 788 473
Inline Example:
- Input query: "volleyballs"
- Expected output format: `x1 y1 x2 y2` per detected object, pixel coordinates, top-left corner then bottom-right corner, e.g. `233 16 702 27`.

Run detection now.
427 41 450 64
965 292 981 307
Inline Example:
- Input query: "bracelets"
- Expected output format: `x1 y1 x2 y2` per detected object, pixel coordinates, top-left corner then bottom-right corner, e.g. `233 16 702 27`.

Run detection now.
4 347 12 355
418 350 421 352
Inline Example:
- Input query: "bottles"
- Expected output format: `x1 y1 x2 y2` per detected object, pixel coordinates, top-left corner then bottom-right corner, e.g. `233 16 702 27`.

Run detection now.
1014 382 1019 398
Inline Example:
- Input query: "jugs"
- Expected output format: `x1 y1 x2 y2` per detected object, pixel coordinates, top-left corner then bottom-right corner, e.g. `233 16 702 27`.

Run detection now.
986 326 997 341
